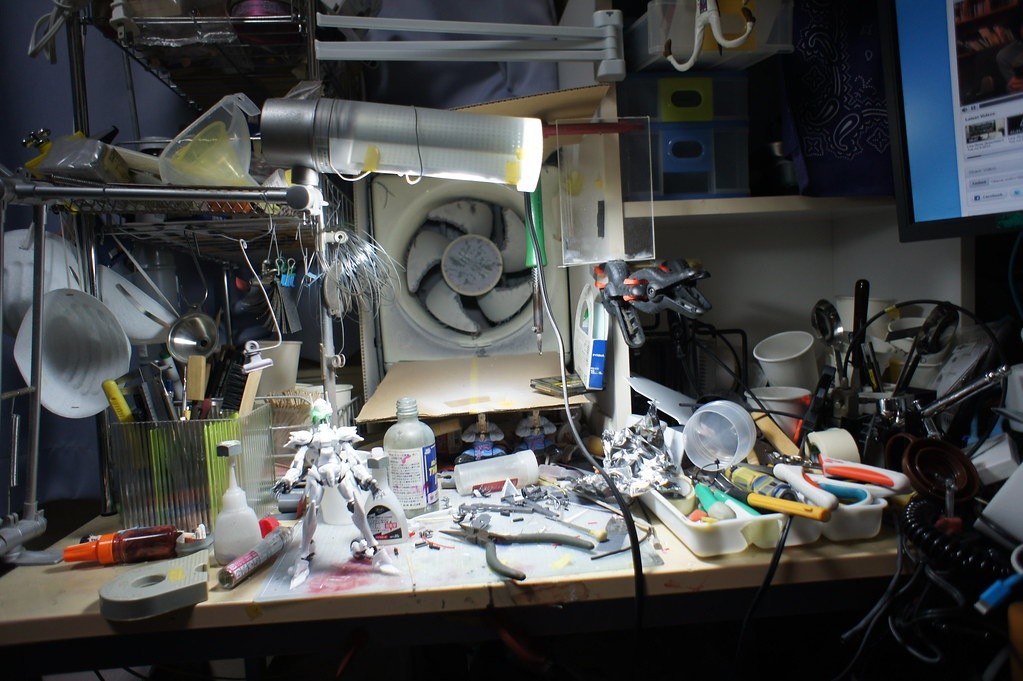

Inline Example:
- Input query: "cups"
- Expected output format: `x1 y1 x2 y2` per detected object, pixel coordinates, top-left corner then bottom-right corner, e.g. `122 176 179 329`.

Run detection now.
744 386 811 440
681 399 756 472
753 330 819 396
254 340 352 420
454 449 539 495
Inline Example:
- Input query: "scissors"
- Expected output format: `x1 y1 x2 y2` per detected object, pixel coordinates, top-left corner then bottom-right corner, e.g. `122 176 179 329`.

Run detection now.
735 463 897 513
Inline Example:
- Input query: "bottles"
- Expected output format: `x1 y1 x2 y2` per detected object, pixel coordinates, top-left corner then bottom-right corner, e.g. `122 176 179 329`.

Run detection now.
63 526 176 565
213 454 262 566
363 447 408 547
383 397 440 519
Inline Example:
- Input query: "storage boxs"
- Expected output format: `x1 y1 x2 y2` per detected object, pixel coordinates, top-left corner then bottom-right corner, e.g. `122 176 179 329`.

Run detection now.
618 0 795 202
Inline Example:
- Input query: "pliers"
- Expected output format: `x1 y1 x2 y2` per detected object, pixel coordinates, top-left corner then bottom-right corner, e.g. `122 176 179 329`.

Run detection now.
439 523 595 581
757 440 909 511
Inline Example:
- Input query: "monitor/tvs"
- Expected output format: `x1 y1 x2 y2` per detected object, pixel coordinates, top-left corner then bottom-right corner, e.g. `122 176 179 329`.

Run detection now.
882 0 1023 245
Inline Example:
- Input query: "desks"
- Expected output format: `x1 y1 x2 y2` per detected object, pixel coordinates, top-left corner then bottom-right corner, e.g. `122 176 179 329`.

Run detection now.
0 457 903 632
